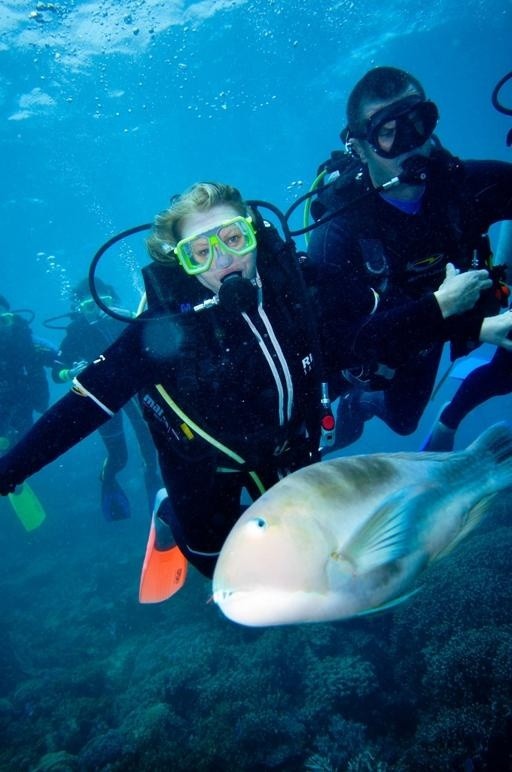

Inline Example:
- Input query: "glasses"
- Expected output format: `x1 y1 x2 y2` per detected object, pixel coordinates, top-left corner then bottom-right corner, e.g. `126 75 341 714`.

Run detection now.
349 94 440 160
80 296 114 316
0 312 15 330
174 214 258 277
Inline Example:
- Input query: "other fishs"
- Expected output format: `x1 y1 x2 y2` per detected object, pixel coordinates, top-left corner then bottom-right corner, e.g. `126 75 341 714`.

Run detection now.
210 415 511 629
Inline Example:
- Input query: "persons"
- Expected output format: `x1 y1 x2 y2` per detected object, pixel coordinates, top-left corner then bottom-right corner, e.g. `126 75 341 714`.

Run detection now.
424 329 510 453
51 276 164 523
1 183 511 583
1 297 59 532
304 67 512 434
